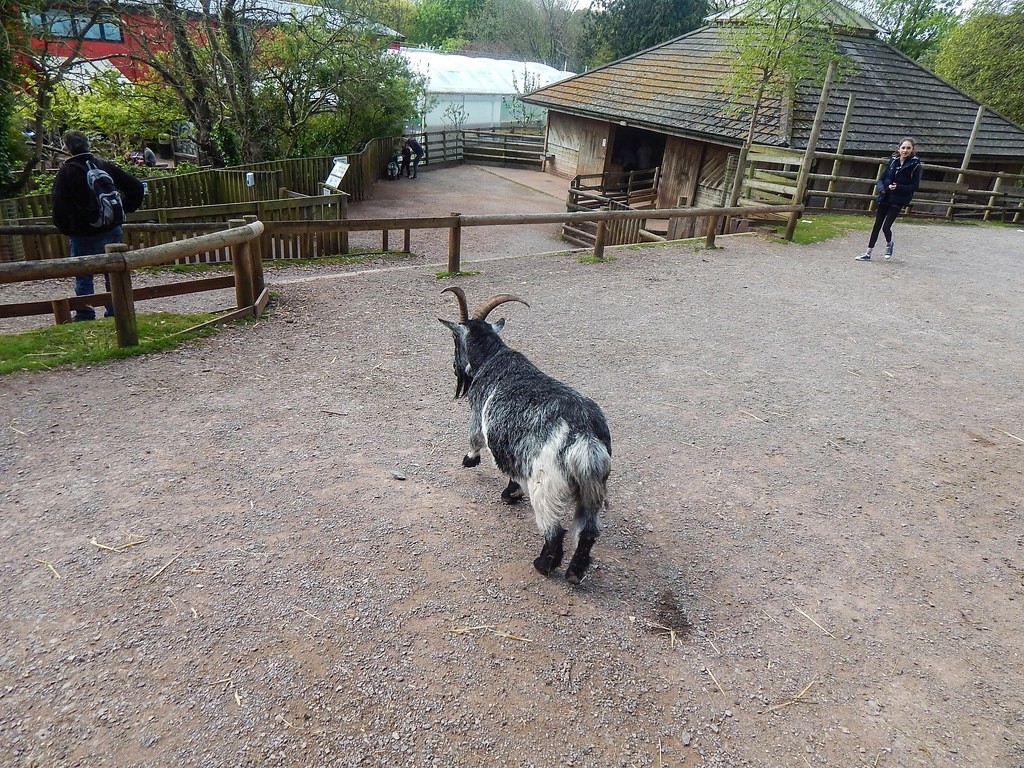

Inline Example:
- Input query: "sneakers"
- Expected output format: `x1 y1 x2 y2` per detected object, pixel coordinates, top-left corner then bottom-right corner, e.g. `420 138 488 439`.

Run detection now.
855 252 871 261
884 243 894 259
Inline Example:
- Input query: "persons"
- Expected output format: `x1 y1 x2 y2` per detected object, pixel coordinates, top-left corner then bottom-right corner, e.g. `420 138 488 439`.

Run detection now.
398 141 411 177
140 143 156 168
405 139 424 179
50 130 144 322
854 137 921 261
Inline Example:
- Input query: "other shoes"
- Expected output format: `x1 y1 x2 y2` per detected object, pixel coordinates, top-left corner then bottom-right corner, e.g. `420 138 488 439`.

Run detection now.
72 315 95 322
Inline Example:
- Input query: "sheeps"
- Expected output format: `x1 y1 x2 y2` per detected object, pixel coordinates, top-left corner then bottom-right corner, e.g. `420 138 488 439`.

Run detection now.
436 286 612 586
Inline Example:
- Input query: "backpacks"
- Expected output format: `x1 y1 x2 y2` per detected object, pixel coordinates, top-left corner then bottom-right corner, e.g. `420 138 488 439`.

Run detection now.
68 159 127 228
